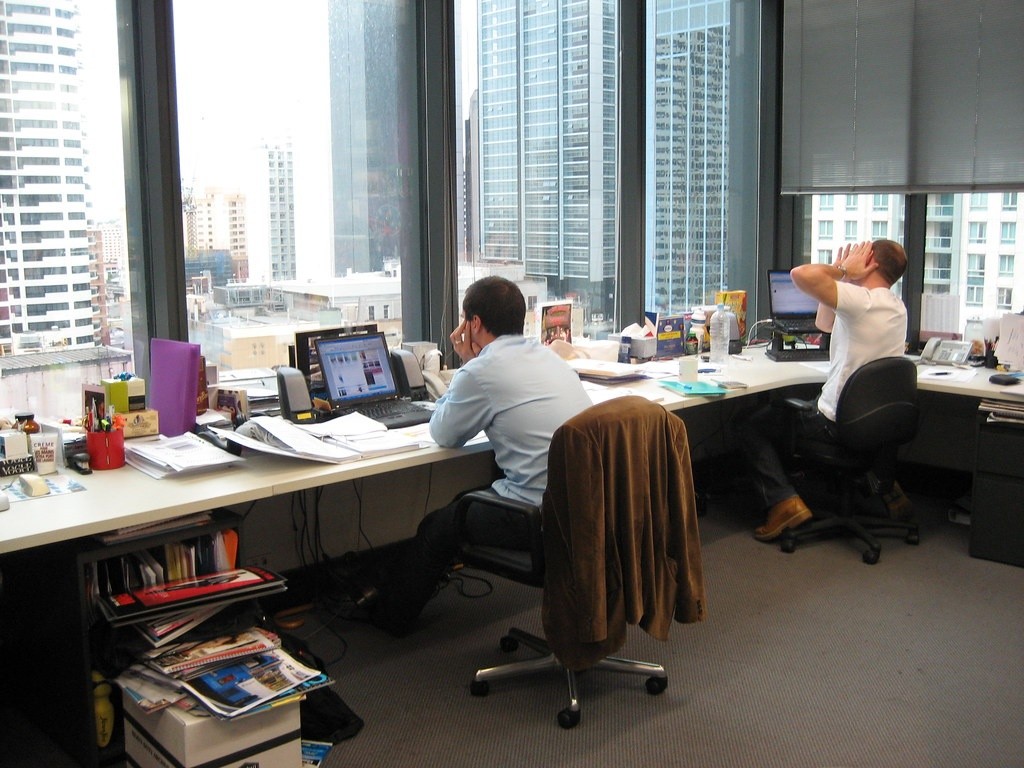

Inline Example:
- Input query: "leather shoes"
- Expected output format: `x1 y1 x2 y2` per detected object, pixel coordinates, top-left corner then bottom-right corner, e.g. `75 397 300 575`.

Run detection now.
880 481 915 522
753 496 813 541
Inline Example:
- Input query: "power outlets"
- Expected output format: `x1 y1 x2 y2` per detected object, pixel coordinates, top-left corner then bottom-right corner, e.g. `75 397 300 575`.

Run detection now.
245 553 274 571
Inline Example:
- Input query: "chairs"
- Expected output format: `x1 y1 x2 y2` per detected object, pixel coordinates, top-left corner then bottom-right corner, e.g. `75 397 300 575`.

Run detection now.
462 396 704 730
774 357 920 565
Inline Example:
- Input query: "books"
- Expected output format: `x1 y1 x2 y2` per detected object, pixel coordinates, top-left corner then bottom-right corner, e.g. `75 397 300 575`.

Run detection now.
710 377 749 389
250 411 420 464
82 508 335 721
565 359 643 384
301 740 333 768
978 398 1024 423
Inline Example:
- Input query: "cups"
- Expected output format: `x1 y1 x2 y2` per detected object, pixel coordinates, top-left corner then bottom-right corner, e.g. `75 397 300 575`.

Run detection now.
678 357 698 384
86 427 125 470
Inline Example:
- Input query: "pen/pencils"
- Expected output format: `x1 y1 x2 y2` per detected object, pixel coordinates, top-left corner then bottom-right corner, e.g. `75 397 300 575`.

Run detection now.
929 371 952 376
984 336 998 351
676 382 692 390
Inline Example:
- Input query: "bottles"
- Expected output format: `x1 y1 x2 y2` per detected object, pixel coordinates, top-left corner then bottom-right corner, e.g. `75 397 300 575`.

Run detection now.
686 332 698 355
689 310 706 354
710 303 730 364
11 412 40 454
964 319 985 361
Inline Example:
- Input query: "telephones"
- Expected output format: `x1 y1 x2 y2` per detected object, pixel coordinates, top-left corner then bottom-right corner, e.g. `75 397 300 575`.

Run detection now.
920 337 973 366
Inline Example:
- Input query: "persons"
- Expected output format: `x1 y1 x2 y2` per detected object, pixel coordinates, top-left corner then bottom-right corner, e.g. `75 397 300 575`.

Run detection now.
344 277 591 635
731 241 913 541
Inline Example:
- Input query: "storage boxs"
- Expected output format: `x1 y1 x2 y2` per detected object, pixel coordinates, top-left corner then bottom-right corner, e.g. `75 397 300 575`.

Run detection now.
716 290 747 339
656 315 684 357
124 686 302 768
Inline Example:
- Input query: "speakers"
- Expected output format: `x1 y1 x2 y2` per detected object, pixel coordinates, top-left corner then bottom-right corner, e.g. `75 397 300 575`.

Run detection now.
391 349 428 401
277 367 316 425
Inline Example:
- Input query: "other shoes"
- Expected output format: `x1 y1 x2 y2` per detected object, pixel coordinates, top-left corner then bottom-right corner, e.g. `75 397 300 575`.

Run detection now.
326 590 416 640
326 556 380 609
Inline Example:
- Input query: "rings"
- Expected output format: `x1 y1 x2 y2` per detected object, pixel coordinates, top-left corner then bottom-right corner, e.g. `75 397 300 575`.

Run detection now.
455 340 462 345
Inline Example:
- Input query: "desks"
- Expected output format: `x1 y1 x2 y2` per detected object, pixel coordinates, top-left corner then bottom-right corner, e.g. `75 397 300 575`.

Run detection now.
0 337 1024 768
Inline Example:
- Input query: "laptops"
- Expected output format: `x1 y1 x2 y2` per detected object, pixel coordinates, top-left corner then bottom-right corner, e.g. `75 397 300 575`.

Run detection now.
766 269 827 334
313 330 436 430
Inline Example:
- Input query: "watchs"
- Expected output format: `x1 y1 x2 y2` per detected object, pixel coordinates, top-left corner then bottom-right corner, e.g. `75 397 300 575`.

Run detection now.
837 265 847 280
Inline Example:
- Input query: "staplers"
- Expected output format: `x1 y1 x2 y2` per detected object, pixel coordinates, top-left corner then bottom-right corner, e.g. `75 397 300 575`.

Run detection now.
67 452 93 475
